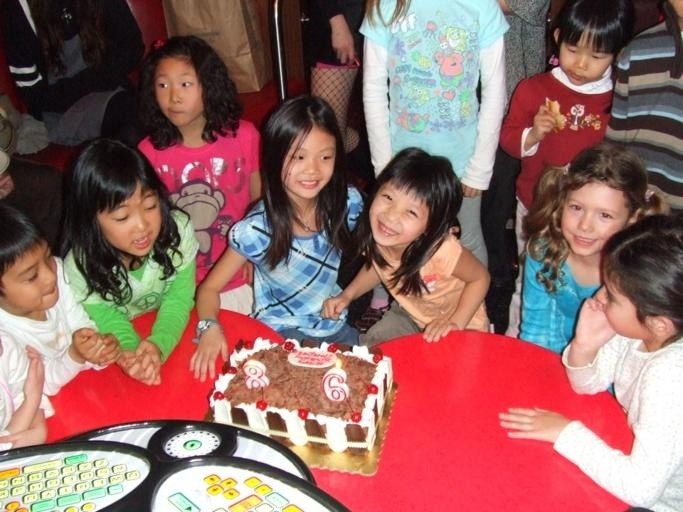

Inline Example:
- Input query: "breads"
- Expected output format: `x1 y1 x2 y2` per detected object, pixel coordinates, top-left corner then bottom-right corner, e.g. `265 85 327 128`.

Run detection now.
545 99 567 134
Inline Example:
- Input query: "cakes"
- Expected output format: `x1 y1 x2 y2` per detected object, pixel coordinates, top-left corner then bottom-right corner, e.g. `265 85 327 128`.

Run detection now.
211 338 400 474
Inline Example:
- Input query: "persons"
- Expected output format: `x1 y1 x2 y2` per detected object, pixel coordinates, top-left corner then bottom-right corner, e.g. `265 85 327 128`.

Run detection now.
319 147 491 344
498 0 614 338
479 0 545 333
513 142 669 398
1 206 125 396
1 331 48 449
309 1 364 155
497 214 681 510
60 139 197 387
188 95 366 382
134 35 264 319
4 0 149 147
358 1 511 273
601 0 681 230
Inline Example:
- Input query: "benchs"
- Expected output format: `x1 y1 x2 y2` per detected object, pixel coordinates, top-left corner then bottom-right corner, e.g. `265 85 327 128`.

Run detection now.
0 2 309 176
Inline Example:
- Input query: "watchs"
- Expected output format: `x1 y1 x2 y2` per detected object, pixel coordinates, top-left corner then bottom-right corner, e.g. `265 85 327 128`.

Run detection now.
191 319 219 344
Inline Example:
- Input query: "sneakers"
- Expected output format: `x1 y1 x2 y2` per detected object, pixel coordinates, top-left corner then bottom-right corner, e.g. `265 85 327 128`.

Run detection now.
355 304 391 333
